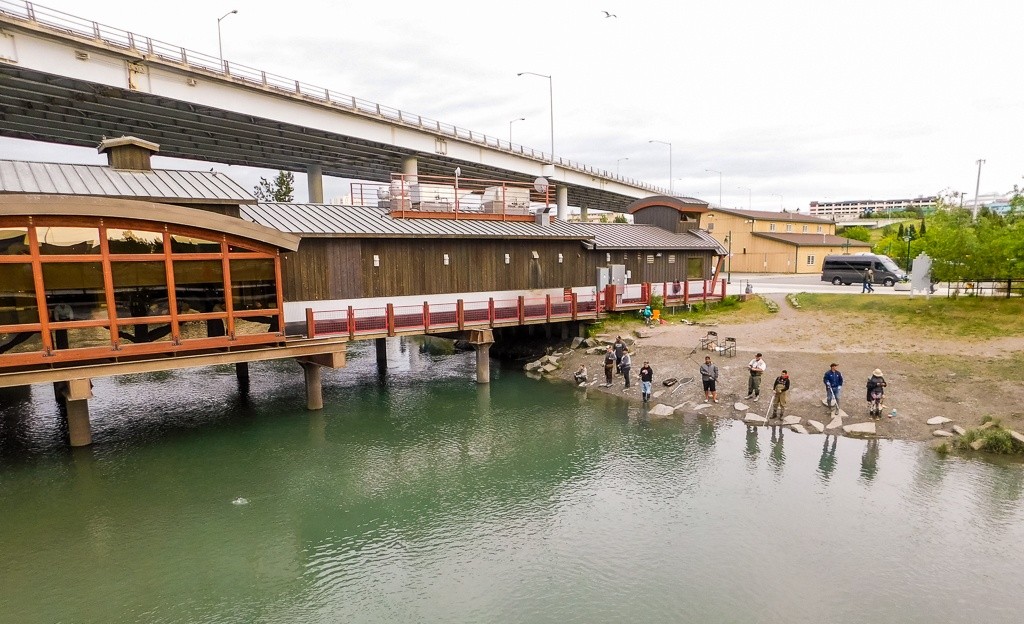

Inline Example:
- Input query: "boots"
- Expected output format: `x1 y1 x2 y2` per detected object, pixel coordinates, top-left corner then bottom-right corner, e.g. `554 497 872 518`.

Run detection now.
643 393 650 402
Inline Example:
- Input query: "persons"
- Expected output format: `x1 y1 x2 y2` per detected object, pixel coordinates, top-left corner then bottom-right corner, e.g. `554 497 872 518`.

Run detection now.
823 363 844 415
699 356 719 403
638 361 653 402
861 268 875 293
769 370 791 421
573 364 587 385
612 335 629 374
866 368 887 420
617 348 631 391
745 353 766 402
603 345 617 387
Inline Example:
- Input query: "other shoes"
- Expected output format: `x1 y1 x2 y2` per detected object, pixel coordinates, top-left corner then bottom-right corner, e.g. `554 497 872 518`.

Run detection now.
876 416 880 420
704 399 709 402
868 415 872 419
744 394 753 399
826 410 832 413
835 410 839 415
872 290 874 293
713 399 718 402
780 414 783 421
754 395 759 401
868 289 871 292
861 291 865 293
769 413 777 419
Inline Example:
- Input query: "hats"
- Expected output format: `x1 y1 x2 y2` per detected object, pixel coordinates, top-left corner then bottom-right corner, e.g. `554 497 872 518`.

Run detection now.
830 362 839 366
864 267 868 270
873 368 884 377
606 345 611 349
755 353 763 357
622 347 628 351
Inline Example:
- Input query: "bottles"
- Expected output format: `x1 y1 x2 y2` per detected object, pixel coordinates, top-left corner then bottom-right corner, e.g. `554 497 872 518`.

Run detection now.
893 409 896 416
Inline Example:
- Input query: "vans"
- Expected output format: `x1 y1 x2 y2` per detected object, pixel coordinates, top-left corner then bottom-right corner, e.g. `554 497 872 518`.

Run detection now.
821 254 905 287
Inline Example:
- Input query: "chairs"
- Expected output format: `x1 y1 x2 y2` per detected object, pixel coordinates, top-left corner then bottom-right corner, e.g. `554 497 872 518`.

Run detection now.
650 310 660 324
702 331 718 352
720 337 736 358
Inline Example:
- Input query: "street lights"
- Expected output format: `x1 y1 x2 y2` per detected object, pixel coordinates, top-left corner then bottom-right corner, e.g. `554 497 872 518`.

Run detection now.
217 9 237 74
705 169 722 206
517 72 554 161
617 157 628 175
509 117 525 146
649 140 672 190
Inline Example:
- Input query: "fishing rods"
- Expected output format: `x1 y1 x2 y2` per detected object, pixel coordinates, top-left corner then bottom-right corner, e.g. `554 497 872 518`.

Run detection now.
828 385 840 412
762 394 776 430
687 354 720 384
844 260 875 287
